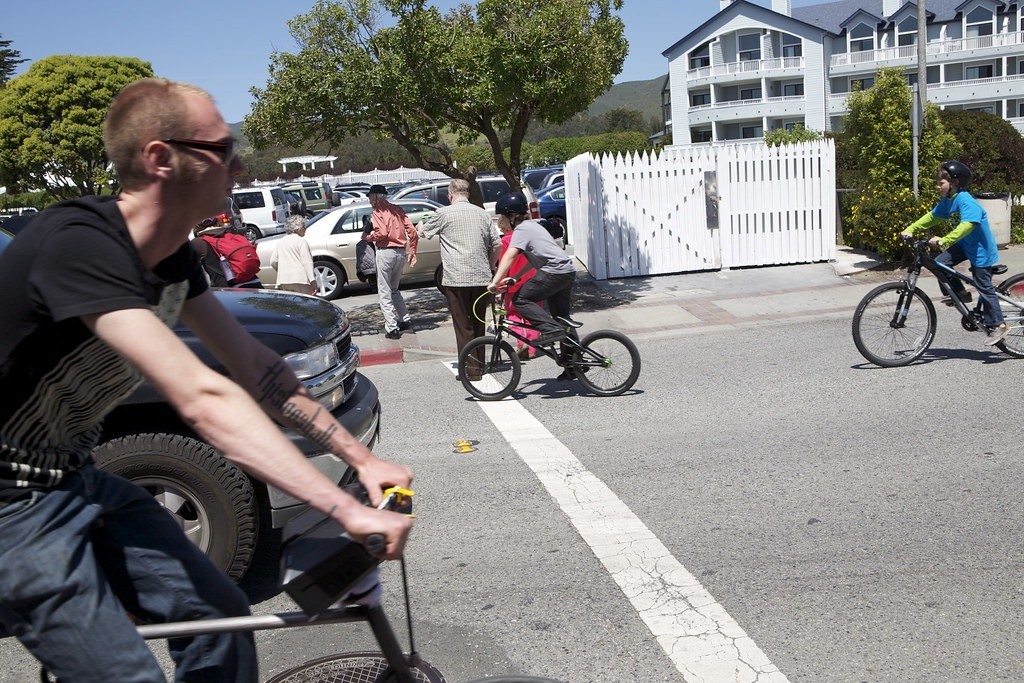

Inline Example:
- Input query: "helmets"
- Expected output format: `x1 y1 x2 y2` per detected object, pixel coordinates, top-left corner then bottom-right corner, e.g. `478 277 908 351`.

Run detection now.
193 198 246 235
496 192 528 216
941 159 972 187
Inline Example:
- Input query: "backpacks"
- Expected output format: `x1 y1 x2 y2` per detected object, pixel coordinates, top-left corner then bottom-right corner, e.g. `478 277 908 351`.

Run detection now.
207 228 260 283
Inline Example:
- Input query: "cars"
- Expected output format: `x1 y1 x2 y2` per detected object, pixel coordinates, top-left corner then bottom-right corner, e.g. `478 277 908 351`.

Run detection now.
200 162 570 253
247 199 449 299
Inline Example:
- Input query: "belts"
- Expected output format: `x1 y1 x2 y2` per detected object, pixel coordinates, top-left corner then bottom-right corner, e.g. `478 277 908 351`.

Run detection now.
378 247 405 249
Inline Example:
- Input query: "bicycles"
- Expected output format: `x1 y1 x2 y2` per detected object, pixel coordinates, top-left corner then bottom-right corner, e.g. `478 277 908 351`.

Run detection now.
44 473 571 683
456 275 642 401
851 235 1024 369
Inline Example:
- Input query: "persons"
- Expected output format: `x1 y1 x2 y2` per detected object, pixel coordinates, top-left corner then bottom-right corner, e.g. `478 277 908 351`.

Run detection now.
0 77 417 682
271 214 318 296
416 179 503 381
494 214 544 360
362 187 419 340
903 160 1011 346
191 215 266 290
487 191 590 381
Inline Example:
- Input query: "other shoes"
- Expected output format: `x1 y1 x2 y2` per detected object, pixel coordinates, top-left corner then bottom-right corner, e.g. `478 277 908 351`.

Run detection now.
456 373 480 381
515 344 529 357
398 319 413 331
556 364 591 382
386 329 399 339
529 328 566 346
940 291 973 303
527 352 537 359
982 323 1011 346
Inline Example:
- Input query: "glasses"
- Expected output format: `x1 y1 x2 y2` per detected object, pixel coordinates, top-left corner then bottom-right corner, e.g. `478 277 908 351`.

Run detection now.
141 137 237 165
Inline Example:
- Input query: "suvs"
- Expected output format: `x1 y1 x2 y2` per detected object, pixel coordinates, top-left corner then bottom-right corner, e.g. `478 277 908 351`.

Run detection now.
0 226 383 624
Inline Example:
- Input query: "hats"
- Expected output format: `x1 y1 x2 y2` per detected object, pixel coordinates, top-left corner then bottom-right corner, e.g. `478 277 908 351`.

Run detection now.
366 184 389 196
